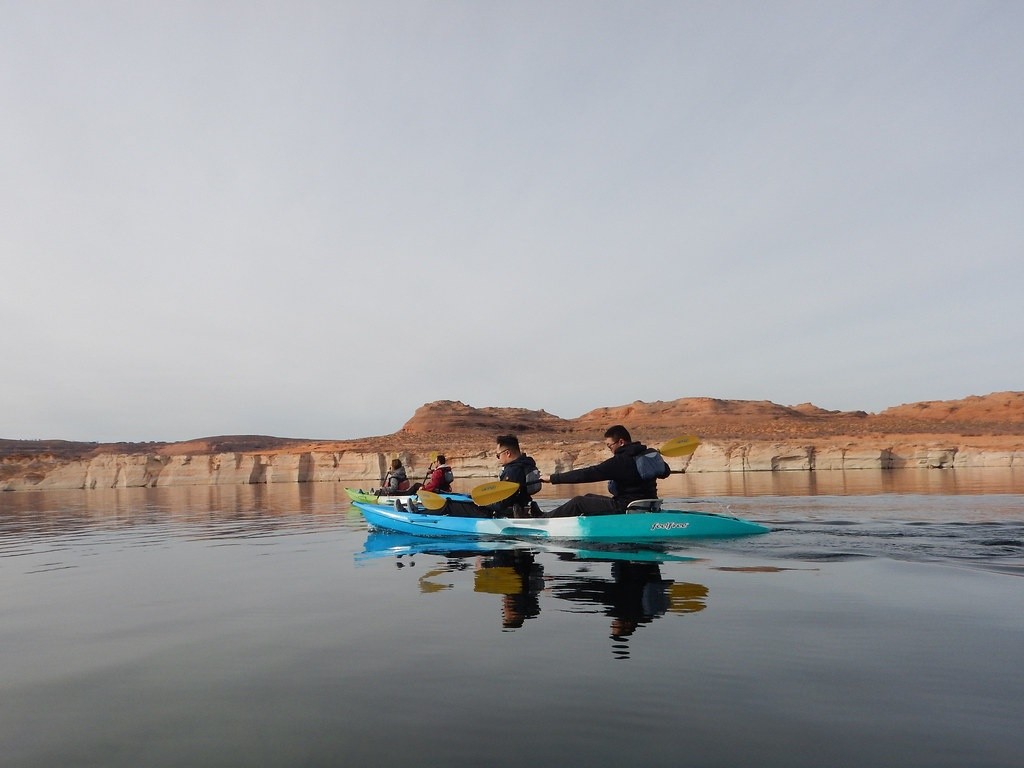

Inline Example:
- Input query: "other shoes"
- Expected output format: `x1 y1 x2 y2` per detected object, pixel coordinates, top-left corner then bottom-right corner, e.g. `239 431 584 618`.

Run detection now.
407 498 420 513
530 501 544 517
394 499 408 512
370 488 374 494
512 504 525 518
358 489 365 495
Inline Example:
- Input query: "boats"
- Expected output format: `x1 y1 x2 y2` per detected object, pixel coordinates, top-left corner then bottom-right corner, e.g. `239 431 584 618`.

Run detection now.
345 485 474 506
352 502 770 540
354 526 712 564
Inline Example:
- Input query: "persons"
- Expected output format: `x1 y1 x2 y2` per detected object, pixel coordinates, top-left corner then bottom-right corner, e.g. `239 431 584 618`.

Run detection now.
394 434 541 518
359 459 410 496
396 455 454 496
531 425 671 518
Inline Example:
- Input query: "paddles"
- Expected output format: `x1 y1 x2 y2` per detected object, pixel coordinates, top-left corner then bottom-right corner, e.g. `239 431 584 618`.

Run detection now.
422 450 438 487
416 490 474 510
372 453 398 504
471 432 702 506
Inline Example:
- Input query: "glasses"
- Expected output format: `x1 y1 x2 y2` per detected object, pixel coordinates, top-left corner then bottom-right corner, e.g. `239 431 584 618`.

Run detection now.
496 449 508 459
434 461 438 464
608 440 619 450
